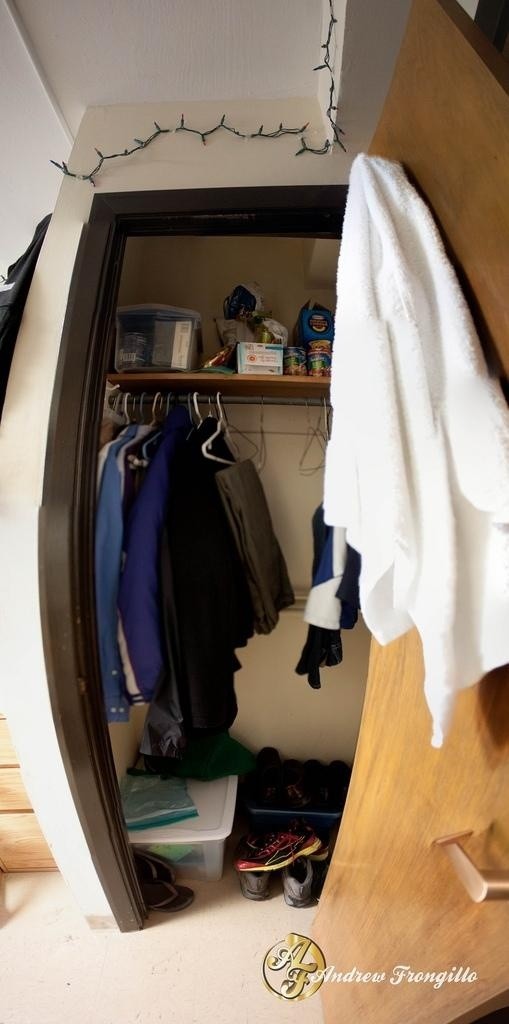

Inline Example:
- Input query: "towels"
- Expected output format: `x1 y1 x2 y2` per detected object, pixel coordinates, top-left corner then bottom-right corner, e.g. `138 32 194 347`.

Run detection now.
322 153 509 750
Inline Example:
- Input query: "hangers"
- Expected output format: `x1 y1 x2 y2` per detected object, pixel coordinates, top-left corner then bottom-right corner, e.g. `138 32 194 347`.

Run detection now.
109 392 332 475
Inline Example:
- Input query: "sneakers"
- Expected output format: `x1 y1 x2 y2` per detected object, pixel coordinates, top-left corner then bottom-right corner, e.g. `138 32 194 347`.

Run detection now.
234 821 330 908
249 746 349 812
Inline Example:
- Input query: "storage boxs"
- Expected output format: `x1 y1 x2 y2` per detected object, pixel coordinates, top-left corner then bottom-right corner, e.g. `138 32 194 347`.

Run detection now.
128 775 238 882
114 303 203 374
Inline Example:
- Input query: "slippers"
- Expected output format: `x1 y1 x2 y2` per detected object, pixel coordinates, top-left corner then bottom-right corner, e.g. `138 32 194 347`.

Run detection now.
133 850 195 912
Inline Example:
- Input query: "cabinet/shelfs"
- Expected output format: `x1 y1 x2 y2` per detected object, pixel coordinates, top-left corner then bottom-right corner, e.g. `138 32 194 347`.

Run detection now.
309 0 509 1024
0 714 60 873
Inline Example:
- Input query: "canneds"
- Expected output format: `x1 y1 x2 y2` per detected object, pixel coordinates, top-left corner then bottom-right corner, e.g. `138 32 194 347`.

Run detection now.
283 346 308 375
308 339 331 377
